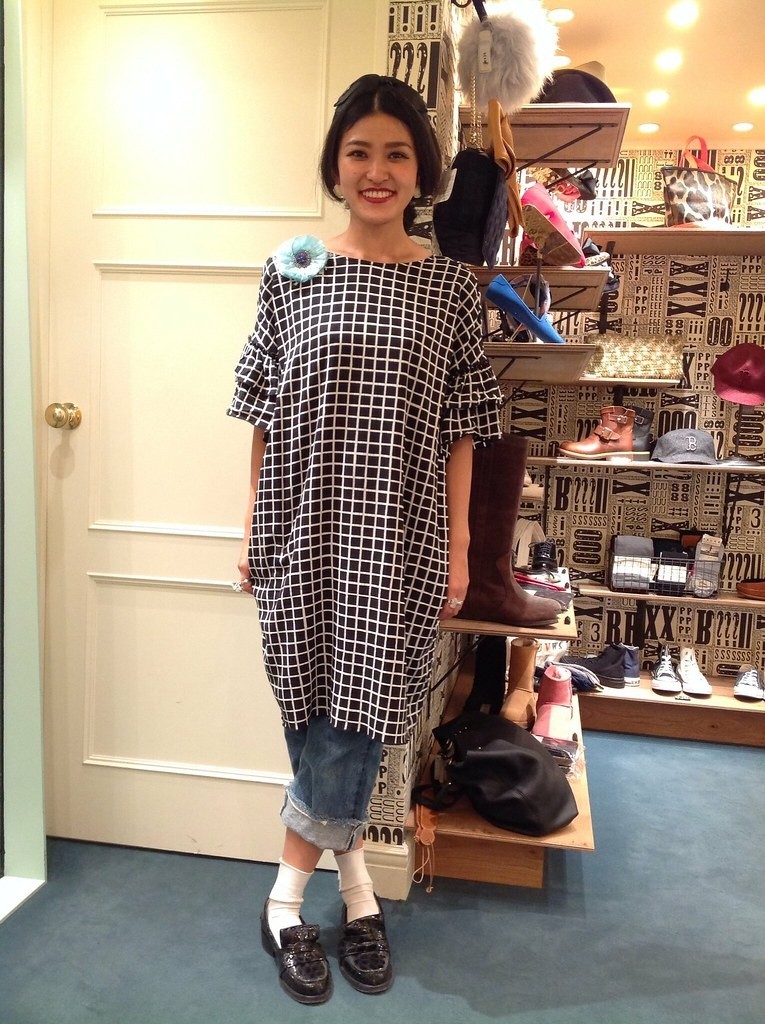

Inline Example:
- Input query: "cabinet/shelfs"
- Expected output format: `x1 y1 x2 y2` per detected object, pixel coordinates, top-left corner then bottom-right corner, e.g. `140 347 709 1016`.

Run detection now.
416 100 765 887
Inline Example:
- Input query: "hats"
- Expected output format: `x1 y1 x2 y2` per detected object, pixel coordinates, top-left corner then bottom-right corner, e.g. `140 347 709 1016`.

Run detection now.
651 428 718 465
711 343 765 406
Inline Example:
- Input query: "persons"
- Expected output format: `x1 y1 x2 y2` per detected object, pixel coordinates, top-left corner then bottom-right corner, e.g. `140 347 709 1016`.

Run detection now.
224 72 505 1007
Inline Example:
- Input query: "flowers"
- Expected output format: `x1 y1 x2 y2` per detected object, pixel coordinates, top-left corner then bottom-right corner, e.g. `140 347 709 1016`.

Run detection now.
275 234 328 282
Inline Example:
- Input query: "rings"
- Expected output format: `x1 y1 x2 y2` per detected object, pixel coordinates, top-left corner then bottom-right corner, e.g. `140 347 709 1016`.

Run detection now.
446 598 464 608
232 578 252 593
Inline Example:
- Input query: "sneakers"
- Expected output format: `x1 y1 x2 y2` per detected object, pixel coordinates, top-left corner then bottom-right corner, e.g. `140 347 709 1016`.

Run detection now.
560 642 626 689
546 660 600 691
615 643 641 687
651 642 683 692
733 663 763 700
675 644 713 695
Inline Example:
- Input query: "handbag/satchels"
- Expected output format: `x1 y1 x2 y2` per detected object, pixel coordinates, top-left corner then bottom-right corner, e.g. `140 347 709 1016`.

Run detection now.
661 135 738 227
578 330 688 379
411 709 579 836
433 22 507 271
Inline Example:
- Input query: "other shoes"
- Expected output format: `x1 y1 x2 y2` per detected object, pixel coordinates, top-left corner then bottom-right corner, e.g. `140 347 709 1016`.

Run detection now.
625 404 654 460
549 164 597 200
520 183 585 268
737 579 765 601
485 273 566 343
528 538 557 572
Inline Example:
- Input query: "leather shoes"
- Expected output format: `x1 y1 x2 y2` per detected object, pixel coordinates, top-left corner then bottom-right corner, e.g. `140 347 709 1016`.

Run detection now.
260 898 331 1004
338 891 394 994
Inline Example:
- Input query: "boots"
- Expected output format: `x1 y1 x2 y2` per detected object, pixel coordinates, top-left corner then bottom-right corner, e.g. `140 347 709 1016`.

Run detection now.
556 405 636 463
499 637 536 730
532 665 573 741
456 436 562 626
